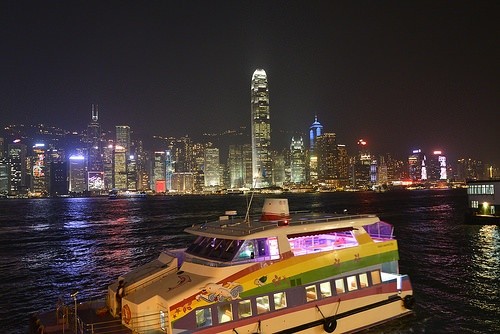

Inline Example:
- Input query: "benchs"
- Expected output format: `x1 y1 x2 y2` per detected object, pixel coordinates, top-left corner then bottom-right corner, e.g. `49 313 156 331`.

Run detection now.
218 279 366 323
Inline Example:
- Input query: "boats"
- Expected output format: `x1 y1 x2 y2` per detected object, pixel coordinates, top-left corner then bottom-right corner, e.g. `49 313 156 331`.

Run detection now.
35 192 415 334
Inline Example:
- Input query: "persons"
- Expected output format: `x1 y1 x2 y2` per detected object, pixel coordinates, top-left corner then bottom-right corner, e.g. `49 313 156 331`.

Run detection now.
116 279 125 315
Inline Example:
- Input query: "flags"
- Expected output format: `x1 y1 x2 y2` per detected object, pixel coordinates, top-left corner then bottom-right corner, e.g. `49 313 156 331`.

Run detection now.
260 161 265 178
